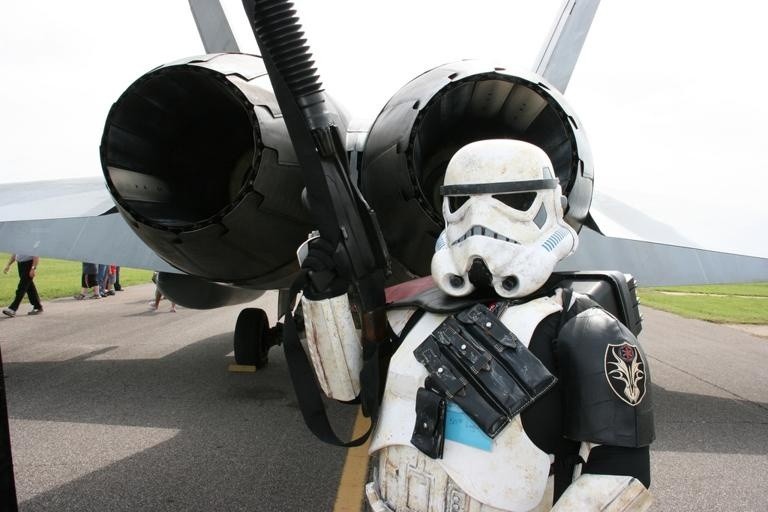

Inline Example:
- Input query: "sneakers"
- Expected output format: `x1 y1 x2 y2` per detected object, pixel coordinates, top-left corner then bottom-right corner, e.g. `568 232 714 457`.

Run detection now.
3 288 124 317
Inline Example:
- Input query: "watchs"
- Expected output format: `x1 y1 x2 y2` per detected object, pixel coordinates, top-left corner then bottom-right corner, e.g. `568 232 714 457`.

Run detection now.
31 265 37 270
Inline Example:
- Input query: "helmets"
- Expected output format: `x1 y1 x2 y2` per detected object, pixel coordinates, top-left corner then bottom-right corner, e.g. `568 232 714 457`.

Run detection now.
430 138 578 299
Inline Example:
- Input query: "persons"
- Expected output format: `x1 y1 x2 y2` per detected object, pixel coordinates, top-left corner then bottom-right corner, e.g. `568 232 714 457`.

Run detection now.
300 137 657 512
147 270 177 314
72 262 124 300
2 252 44 317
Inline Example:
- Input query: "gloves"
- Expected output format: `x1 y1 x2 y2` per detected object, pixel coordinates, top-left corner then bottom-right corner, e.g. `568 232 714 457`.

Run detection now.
296 237 349 301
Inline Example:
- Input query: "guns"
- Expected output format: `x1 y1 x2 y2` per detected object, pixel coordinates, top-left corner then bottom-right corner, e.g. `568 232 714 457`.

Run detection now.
242 1 392 418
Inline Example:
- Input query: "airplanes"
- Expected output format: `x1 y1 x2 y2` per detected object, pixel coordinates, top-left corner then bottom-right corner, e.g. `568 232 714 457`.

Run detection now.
1 1 767 369
